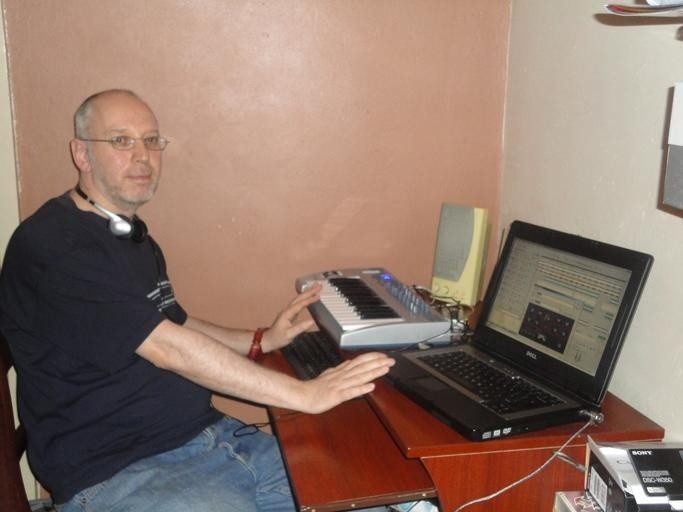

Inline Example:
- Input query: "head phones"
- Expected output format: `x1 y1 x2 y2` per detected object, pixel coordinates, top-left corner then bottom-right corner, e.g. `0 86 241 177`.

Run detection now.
74 183 148 244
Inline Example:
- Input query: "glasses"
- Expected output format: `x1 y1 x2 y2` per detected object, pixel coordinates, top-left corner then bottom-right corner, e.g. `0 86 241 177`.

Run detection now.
80 134 171 152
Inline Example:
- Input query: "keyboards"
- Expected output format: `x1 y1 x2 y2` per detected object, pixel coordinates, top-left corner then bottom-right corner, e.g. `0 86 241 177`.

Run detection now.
280 329 342 383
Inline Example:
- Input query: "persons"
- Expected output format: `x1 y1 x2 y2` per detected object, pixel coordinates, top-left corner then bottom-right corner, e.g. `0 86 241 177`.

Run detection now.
1 88 396 512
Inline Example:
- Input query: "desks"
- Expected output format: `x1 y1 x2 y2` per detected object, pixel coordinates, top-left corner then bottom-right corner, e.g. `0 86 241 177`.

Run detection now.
252 302 666 512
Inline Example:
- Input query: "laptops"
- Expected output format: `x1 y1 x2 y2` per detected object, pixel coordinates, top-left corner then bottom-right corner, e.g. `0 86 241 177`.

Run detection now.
381 219 655 442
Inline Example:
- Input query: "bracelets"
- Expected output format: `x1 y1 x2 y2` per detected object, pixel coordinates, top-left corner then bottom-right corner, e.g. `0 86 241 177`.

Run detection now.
247 325 267 361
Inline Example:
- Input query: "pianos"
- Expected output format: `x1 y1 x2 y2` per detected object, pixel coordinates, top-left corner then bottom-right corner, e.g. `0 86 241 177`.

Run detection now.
296 267 451 351
